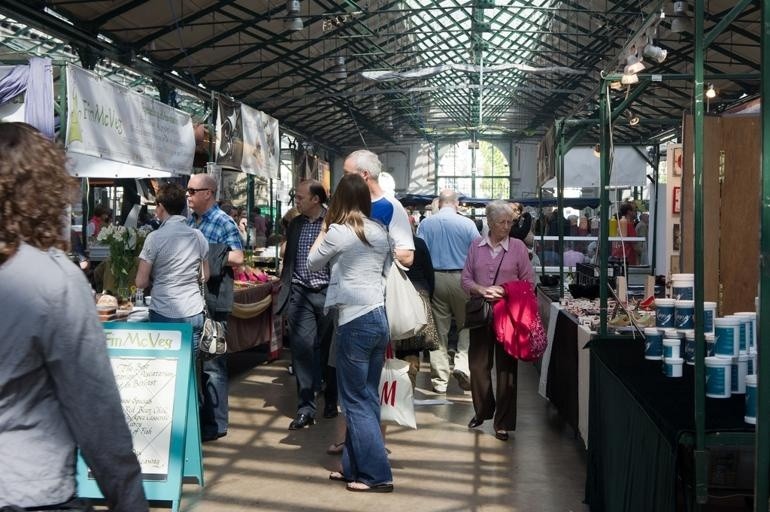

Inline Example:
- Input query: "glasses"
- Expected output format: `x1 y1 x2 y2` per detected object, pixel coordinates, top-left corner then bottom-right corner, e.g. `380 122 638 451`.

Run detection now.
185 188 208 195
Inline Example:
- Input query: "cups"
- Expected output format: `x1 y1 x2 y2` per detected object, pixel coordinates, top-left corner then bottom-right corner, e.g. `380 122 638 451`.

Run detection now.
559 295 620 330
145 296 151 305
117 287 128 302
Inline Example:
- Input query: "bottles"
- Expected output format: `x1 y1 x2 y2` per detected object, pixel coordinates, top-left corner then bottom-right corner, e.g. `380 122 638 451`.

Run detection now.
609 215 617 236
619 216 628 237
579 215 587 235
136 287 143 305
567 264 575 284
590 215 599 236
568 214 578 235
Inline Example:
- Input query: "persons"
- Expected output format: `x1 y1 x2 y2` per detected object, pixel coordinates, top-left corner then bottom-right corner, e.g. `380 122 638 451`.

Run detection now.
304 170 398 494
0 121 152 512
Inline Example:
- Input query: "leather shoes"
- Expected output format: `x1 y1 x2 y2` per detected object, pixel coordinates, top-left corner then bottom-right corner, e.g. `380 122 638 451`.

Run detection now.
496 432 508 440
289 414 314 430
324 402 338 418
468 414 493 428
432 369 471 394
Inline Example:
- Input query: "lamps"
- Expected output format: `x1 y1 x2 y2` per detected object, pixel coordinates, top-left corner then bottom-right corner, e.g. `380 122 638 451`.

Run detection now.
621 0 716 126
284 0 404 138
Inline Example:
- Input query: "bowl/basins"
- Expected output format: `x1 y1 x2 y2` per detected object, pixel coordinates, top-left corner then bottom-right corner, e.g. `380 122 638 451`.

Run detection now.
538 274 561 285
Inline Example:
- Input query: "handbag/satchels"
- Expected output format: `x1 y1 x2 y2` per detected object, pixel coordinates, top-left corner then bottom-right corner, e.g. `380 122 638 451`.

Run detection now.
382 251 428 340
378 343 417 430
199 318 227 360
465 296 494 328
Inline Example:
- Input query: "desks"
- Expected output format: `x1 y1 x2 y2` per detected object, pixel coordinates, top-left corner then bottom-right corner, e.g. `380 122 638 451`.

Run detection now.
534 283 757 511
120 276 284 382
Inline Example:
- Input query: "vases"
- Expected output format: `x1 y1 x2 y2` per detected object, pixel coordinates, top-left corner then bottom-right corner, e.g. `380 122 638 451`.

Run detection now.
117 277 130 300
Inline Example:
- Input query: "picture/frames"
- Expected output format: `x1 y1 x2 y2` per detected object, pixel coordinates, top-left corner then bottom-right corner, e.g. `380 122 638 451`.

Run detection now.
673 187 681 213
673 148 683 177
671 255 680 275
673 224 680 250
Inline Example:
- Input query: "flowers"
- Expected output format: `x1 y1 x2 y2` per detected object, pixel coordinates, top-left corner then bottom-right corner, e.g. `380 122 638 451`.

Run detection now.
94 221 153 276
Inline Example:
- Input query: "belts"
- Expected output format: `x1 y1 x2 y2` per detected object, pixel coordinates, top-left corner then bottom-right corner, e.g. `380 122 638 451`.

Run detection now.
433 269 462 272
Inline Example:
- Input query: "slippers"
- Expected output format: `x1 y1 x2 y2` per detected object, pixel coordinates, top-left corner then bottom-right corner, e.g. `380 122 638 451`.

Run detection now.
328 442 393 492
